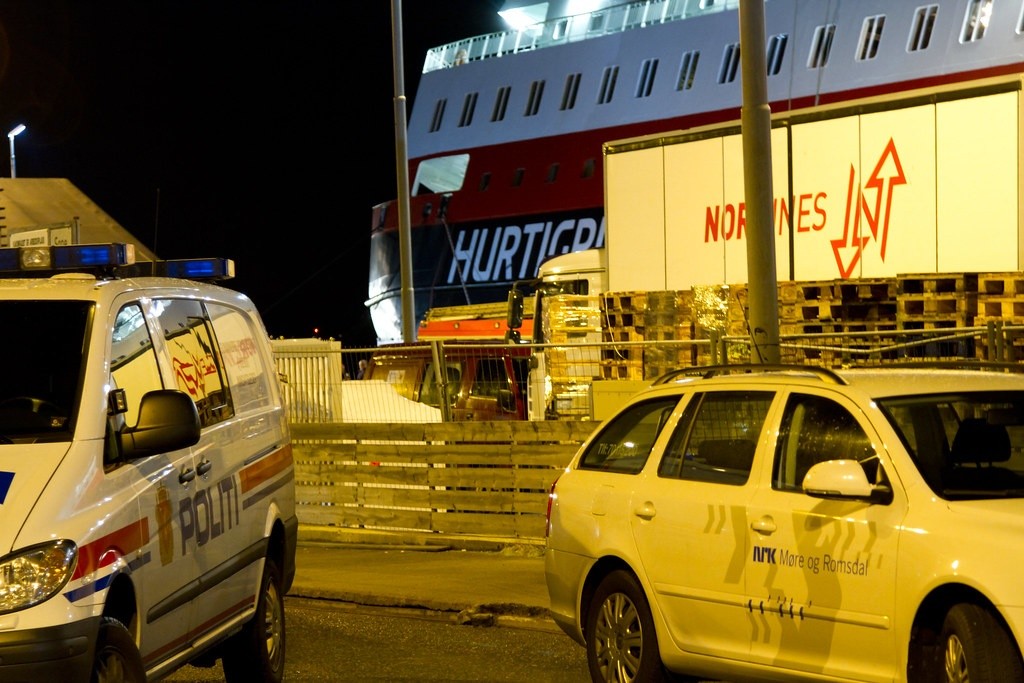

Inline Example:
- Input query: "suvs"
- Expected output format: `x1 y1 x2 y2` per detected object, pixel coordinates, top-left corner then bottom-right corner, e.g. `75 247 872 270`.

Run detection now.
542 362 1023 682
362 342 530 426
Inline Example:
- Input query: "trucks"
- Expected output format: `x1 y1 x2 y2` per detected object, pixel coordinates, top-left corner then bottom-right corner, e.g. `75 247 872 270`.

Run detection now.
506 74 1023 423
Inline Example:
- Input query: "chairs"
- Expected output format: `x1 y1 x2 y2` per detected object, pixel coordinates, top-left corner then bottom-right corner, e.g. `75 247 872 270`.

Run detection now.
949 418 1023 498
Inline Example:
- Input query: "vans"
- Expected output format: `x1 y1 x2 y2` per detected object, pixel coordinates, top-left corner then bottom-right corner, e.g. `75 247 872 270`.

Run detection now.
0 240 294 682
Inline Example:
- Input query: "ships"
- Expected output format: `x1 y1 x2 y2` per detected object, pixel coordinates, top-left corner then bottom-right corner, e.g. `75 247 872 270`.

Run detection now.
364 0 1024 342
